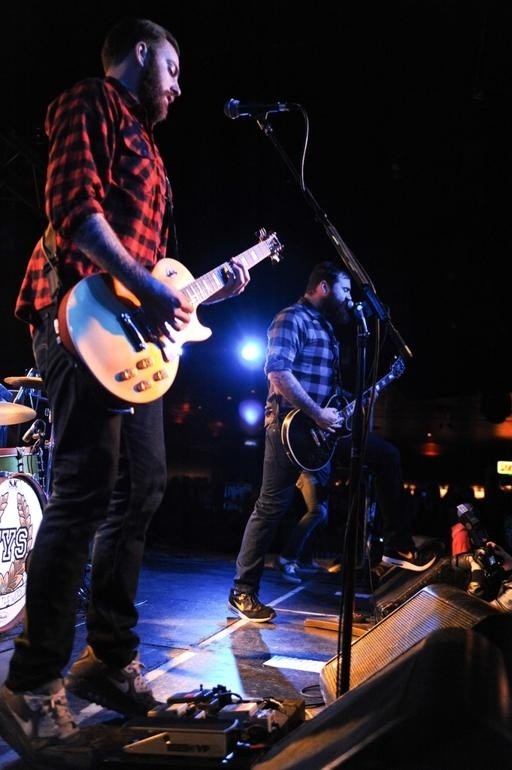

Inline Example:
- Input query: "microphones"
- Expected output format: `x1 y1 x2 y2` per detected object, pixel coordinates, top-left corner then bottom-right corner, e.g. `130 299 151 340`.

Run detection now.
224 98 299 120
347 301 384 310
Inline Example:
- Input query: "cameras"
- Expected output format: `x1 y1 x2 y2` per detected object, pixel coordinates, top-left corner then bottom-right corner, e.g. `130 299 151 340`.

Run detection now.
452 542 504 579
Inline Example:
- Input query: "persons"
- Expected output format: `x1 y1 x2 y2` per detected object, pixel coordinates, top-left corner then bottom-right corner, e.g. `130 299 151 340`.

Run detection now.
464 545 512 612
0 18 251 770
224 260 438 621
279 470 343 585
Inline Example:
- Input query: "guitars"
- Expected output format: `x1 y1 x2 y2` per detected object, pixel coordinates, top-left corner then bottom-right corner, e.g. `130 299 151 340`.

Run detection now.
58 228 282 403
281 354 407 471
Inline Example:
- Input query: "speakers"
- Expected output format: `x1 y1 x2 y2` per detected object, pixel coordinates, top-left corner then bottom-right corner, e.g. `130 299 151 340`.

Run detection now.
248 627 512 770
367 551 455 622
318 584 512 710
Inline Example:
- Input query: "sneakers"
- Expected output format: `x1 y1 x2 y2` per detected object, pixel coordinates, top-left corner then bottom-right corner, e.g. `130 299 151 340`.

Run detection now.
223 585 276 624
298 559 327 574
378 545 439 572
0 675 81 762
274 553 304 585
61 642 161 721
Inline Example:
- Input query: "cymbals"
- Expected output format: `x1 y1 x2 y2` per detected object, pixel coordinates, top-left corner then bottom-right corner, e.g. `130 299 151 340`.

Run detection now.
0 402 36 426
4 377 42 386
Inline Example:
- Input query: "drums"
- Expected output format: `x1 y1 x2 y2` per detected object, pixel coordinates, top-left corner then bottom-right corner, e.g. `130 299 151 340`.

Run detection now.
0 447 40 481
0 470 49 632
